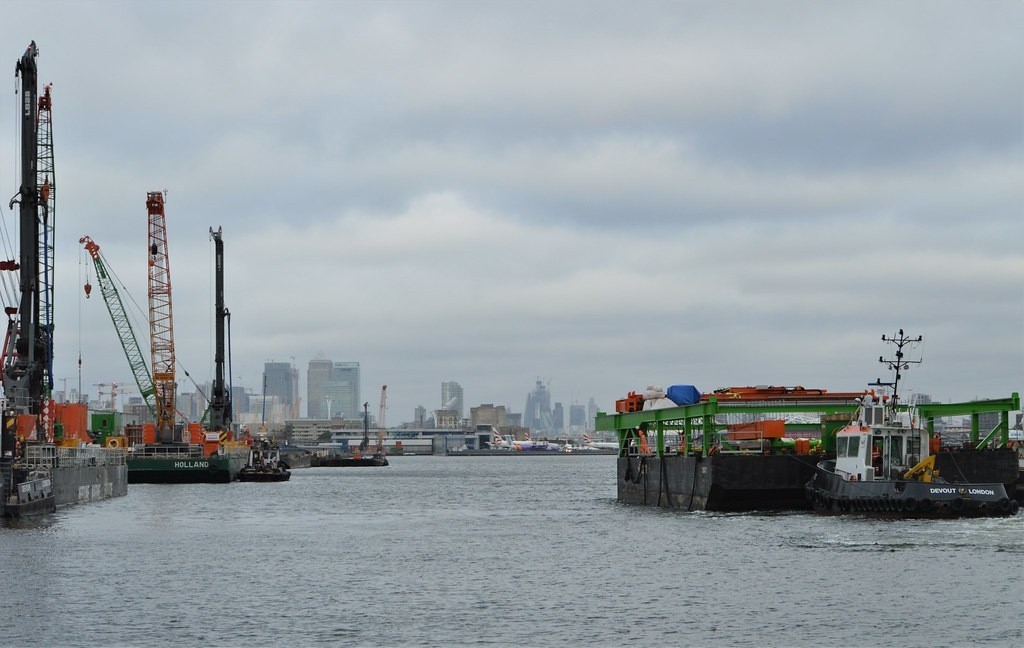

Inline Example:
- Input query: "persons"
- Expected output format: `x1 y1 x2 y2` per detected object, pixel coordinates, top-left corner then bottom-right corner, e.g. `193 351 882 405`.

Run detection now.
871 442 882 475
639 430 651 455
676 428 685 451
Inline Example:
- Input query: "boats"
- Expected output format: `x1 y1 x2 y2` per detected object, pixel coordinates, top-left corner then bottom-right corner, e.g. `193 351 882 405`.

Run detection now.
242 375 291 482
803 328 1019 520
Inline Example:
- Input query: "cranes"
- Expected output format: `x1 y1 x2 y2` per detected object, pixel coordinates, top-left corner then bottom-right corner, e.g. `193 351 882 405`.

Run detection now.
363 386 389 454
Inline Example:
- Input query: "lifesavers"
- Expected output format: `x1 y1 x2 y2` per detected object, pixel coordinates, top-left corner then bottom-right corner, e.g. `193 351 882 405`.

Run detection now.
15 441 21 454
804 478 989 515
109 439 119 449
209 463 219 475
1010 499 1019 515
998 497 1010 511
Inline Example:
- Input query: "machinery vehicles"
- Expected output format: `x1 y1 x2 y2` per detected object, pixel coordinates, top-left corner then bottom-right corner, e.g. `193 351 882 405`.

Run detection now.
0 39 242 464
